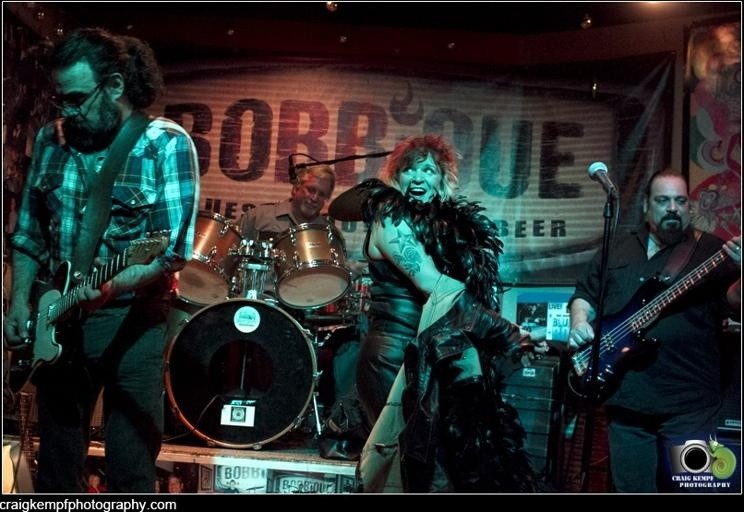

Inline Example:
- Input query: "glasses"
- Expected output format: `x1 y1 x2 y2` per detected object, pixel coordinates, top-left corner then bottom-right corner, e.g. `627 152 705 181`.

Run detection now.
47 75 109 117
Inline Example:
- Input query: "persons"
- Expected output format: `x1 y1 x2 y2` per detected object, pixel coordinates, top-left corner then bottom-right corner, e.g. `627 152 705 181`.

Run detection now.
233 160 368 418
567 167 741 493
353 134 551 492
1 27 203 492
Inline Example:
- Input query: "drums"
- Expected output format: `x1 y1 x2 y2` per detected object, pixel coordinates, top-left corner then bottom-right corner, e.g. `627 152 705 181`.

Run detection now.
303 279 372 328
268 223 352 309
165 297 323 450
174 209 245 306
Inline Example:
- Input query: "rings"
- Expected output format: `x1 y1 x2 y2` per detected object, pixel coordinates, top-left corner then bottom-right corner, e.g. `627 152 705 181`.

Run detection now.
732 246 739 254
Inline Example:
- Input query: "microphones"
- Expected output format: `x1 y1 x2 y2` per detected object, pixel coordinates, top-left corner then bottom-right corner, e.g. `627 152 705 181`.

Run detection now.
588 161 621 201
288 158 298 184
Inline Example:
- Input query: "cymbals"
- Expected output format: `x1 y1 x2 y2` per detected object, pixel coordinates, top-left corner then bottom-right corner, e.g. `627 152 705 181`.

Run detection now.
328 182 370 221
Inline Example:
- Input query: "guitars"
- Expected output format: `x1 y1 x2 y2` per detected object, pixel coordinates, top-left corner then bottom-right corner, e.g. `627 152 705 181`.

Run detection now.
568 249 729 400
8 229 172 395
